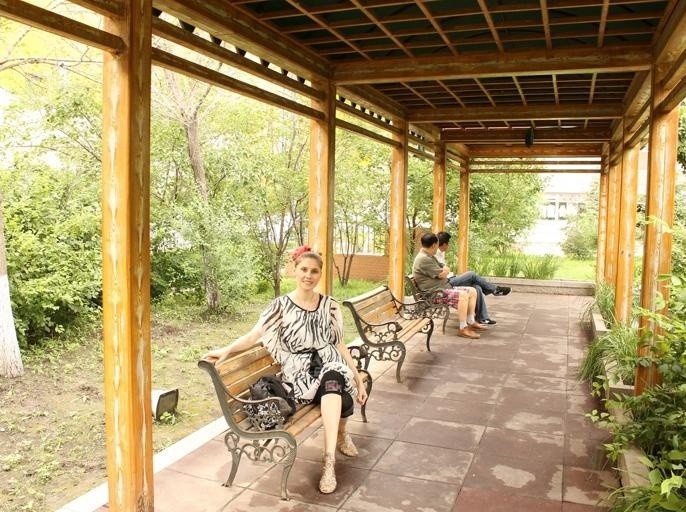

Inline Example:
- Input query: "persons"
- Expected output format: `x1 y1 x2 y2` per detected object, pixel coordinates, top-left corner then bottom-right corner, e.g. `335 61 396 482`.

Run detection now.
199 242 368 495
413 231 489 341
434 231 511 325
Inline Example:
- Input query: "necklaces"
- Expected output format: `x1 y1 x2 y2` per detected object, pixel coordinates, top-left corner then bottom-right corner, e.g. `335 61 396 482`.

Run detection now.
299 294 316 315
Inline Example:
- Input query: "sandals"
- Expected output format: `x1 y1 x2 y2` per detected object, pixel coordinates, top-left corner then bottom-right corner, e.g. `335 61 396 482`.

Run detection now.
319 460 337 494
336 431 359 457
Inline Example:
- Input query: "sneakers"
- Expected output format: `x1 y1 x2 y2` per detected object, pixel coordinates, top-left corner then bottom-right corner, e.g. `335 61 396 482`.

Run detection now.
467 322 489 332
478 319 496 324
457 326 480 339
493 286 510 297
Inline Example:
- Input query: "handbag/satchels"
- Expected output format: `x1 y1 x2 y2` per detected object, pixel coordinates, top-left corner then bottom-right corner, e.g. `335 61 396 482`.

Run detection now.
250 377 294 430
